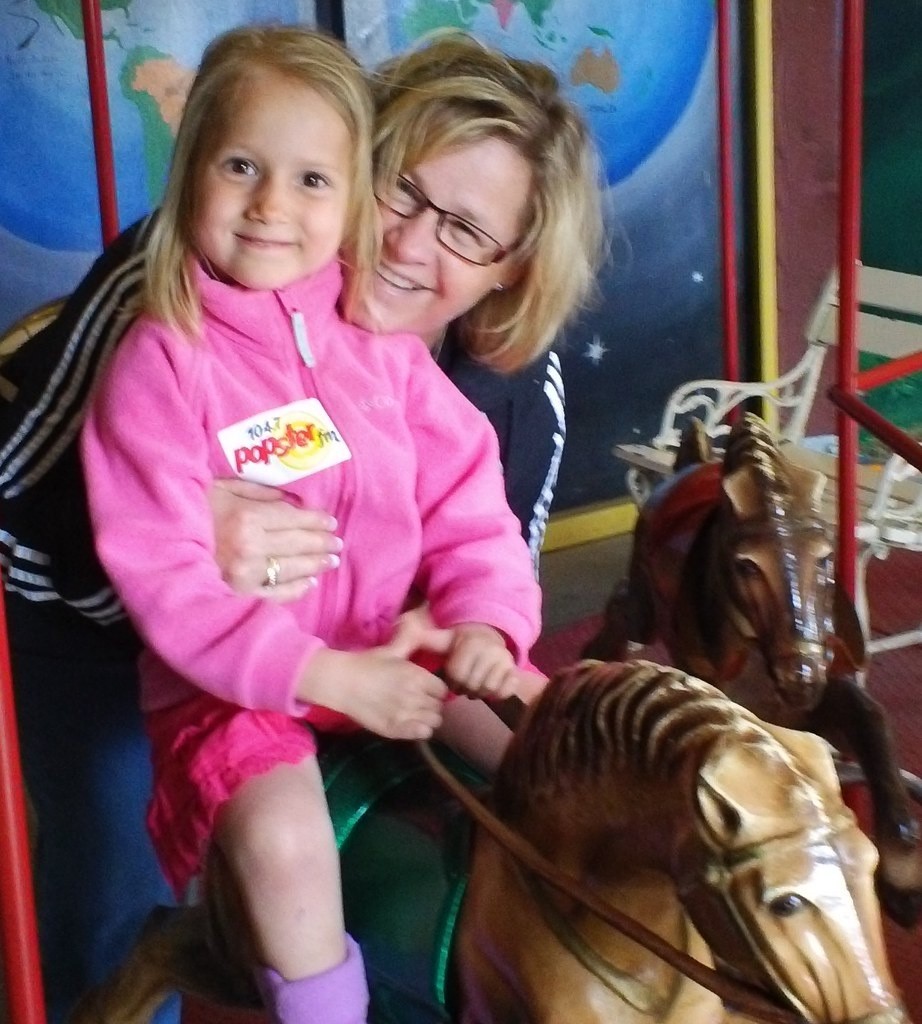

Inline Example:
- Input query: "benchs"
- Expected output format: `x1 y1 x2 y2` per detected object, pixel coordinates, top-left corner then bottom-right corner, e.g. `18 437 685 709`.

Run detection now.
617 256 922 654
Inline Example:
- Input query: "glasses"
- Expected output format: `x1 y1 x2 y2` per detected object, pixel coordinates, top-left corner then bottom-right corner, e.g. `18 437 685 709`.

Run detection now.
369 167 530 268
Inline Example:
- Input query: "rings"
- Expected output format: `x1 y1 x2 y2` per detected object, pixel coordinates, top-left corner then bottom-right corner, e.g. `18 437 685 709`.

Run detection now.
267 555 279 585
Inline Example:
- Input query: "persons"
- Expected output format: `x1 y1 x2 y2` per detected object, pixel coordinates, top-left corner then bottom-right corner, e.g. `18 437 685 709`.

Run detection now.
0 24 608 1024
80 26 549 1024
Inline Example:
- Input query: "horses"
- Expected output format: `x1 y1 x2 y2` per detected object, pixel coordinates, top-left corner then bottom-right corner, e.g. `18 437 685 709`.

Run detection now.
56 658 911 1023
572 409 922 931
